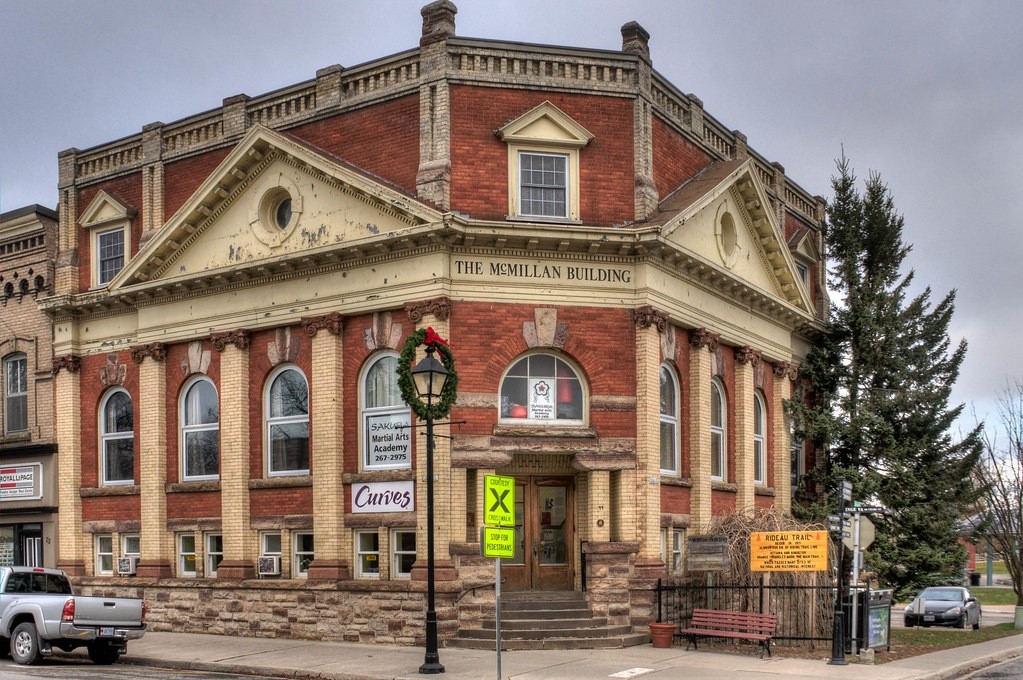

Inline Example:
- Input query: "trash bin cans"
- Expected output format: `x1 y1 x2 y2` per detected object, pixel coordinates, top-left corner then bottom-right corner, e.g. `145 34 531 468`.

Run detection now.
841 587 894 654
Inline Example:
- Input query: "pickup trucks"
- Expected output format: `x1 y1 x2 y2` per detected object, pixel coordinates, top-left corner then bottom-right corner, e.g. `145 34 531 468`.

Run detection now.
0 566 147 667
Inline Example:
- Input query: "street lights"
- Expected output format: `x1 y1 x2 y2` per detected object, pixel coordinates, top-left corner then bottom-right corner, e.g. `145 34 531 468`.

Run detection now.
408 345 445 674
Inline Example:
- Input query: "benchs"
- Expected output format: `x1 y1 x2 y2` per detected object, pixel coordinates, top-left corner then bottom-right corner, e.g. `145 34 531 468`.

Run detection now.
681 609 777 659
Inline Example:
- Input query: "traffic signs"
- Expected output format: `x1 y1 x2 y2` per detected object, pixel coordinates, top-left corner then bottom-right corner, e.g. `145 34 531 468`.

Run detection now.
826 516 841 523
830 525 841 531
842 520 851 527
841 480 854 501
842 531 851 538
844 507 884 513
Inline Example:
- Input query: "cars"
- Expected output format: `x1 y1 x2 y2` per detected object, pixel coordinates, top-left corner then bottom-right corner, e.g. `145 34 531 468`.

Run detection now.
903 585 984 631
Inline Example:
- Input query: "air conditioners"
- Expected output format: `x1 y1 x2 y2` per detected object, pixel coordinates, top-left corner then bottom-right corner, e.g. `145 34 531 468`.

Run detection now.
117 557 139 575
258 556 282 576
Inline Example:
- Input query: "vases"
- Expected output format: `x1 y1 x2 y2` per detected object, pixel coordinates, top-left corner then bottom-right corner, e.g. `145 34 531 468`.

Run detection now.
649 622 677 648
